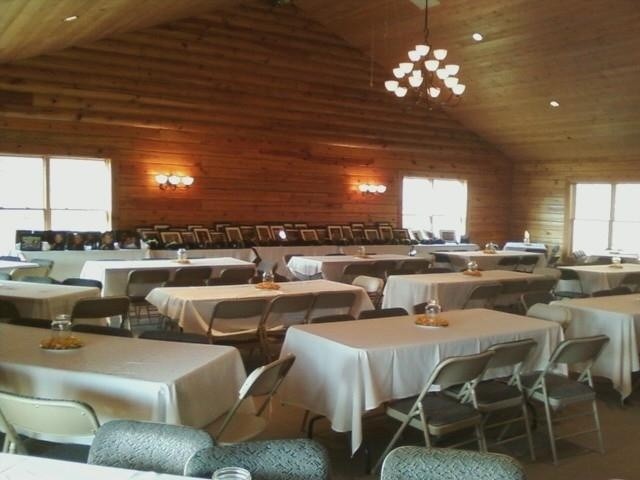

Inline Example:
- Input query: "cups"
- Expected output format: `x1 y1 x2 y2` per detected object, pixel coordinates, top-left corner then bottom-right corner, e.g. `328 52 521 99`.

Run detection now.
611 257 621 265
177 248 188 262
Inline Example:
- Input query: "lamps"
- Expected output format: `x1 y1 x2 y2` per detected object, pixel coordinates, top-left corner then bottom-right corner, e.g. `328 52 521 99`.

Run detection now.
383 0 466 112
359 184 386 198
157 175 194 190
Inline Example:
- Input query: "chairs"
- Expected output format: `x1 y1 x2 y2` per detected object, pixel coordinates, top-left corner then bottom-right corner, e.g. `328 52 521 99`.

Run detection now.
184 439 328 480
381 445 527 480
278 301 612 475
1 241 639 367
1 321 296 456
86 420 215 475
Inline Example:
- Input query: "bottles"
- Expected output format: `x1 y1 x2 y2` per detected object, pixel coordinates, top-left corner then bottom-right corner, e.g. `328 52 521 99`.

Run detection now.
262 269 274 285
524 230 530 246
467 261 478 272
211 466 252 480
356 245 367 259
408 246 416 256
426 299 441 319
50 314 73 338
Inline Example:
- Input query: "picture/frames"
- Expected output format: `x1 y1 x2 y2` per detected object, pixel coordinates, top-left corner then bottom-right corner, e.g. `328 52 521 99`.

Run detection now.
16 224 456 251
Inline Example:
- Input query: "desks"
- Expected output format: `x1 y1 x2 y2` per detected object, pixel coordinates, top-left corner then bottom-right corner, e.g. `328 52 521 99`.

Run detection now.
548 293 639 409
